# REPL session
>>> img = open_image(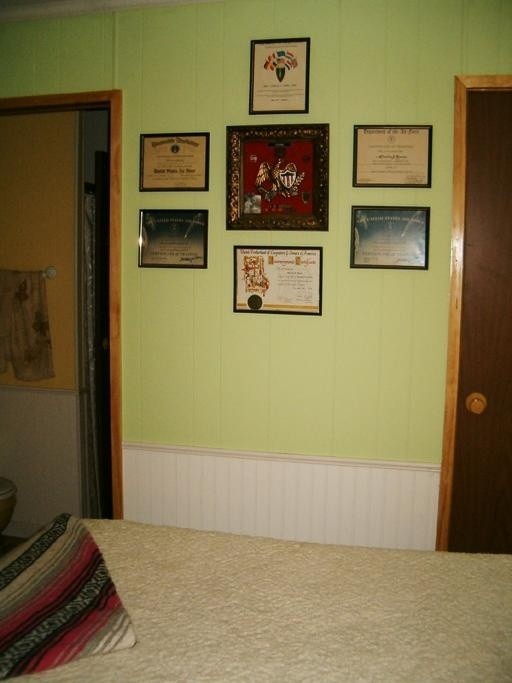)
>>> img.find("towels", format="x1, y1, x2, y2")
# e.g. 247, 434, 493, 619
0, 269, 57, 383
